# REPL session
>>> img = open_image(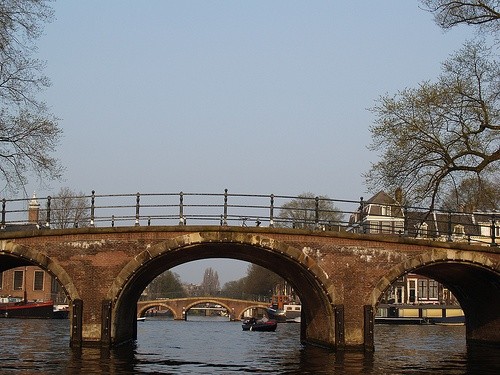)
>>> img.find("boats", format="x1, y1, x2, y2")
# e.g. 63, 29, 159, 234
242, 318, 277, 332
374, 304, 465, 325
0, 267, 70, 320
137, 317, 145, 321
